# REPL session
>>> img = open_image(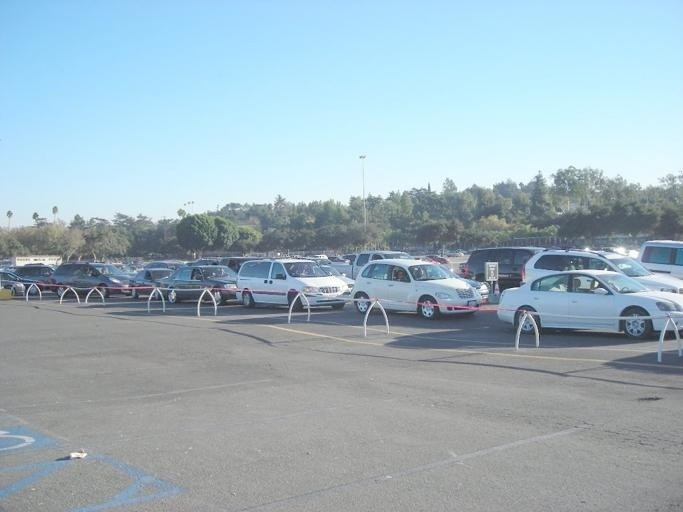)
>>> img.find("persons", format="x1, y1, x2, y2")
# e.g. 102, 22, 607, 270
414, 266, 426, 279
393, 269, 406, 281
595, 278, 621, 295
82, 266, 108, 279
